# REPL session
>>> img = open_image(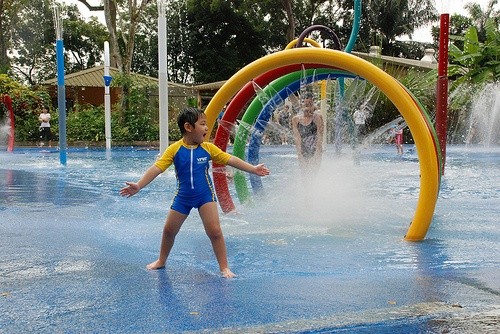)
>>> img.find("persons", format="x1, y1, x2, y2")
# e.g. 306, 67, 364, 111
120, 107, 270, 277
291, 92, 324, 176
392, 121, 403, 155
38, 106, 51, 147
217, 104, 231, 146
277, 107, 291, 146
353, 102, 366, 138
